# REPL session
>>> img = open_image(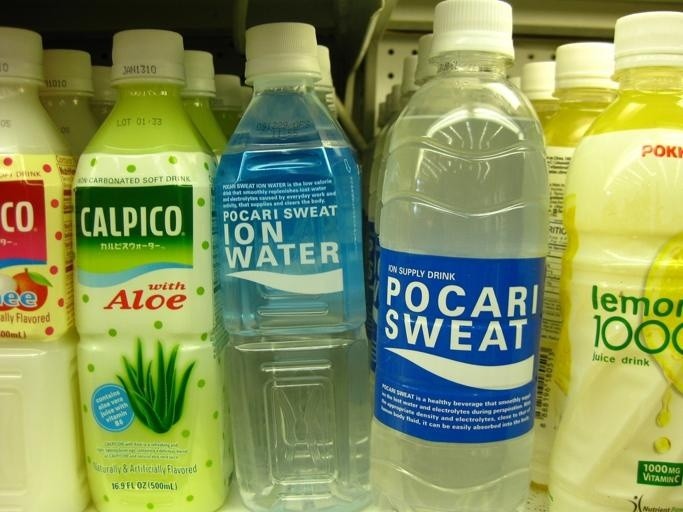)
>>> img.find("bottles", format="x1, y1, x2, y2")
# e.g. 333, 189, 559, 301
0, 0, 683, 512
